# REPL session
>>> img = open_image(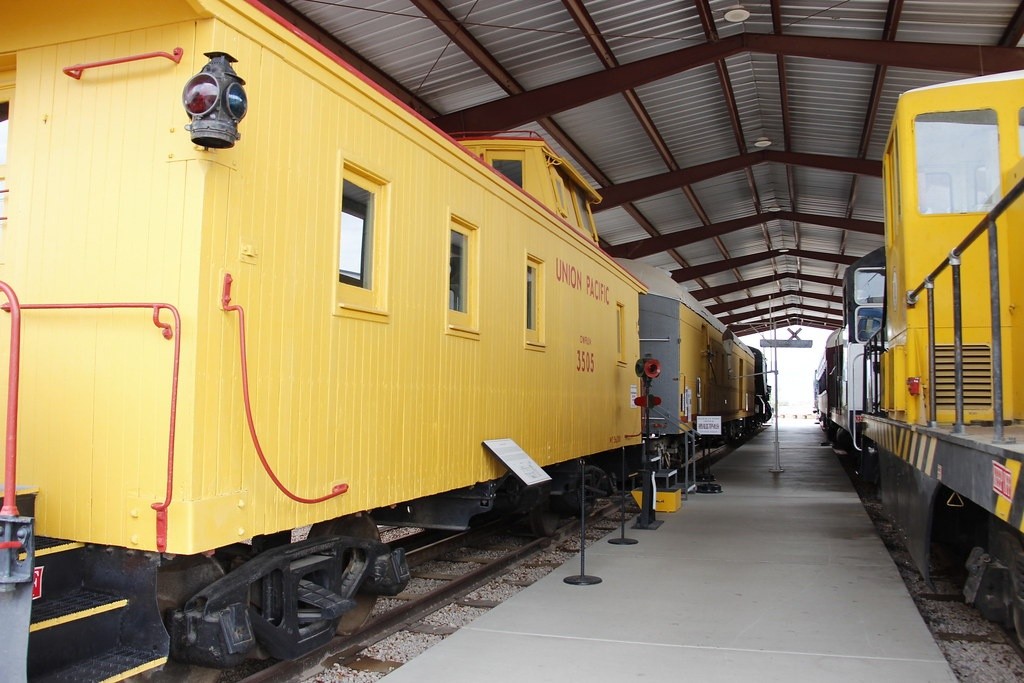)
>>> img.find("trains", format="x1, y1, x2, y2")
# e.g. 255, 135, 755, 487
809, 67, 1022, 653
1, 0, 771, 668
